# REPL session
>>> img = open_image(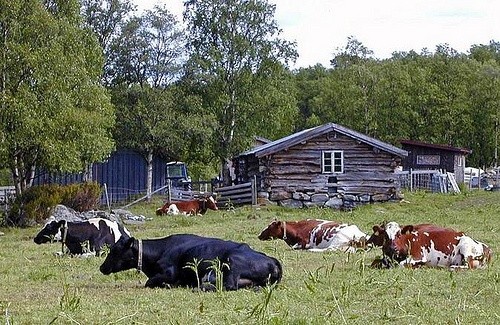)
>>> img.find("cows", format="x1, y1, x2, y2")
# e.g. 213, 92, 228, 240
154, 192, 219, 217
98, 227, 283, 292
257, 216, 371, 254
33, 216, 133, 260
369, 220, 493, 271
